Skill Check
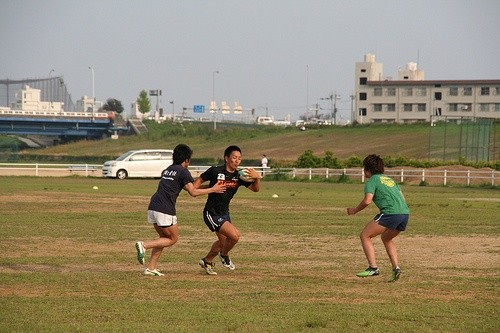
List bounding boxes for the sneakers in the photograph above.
[135,241,146,265]
[199,257,218,275]
[388,266,402,282]
[218,251,236,270]
[143,267,165,277]
[356,267,380,278]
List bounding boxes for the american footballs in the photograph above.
[237,168,256,182]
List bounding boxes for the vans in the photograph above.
[102,149,173,180]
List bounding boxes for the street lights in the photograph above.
[47,69,55,113]
[88,65,94,120]
[306,64,311,121]
[212,71,220,120]
[170,100,175,121]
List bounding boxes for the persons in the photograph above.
[136,143,227,277]
[347,154,409,283]
[261,154,268,168]
[300,126,306,131]
[195,145,261,276]
